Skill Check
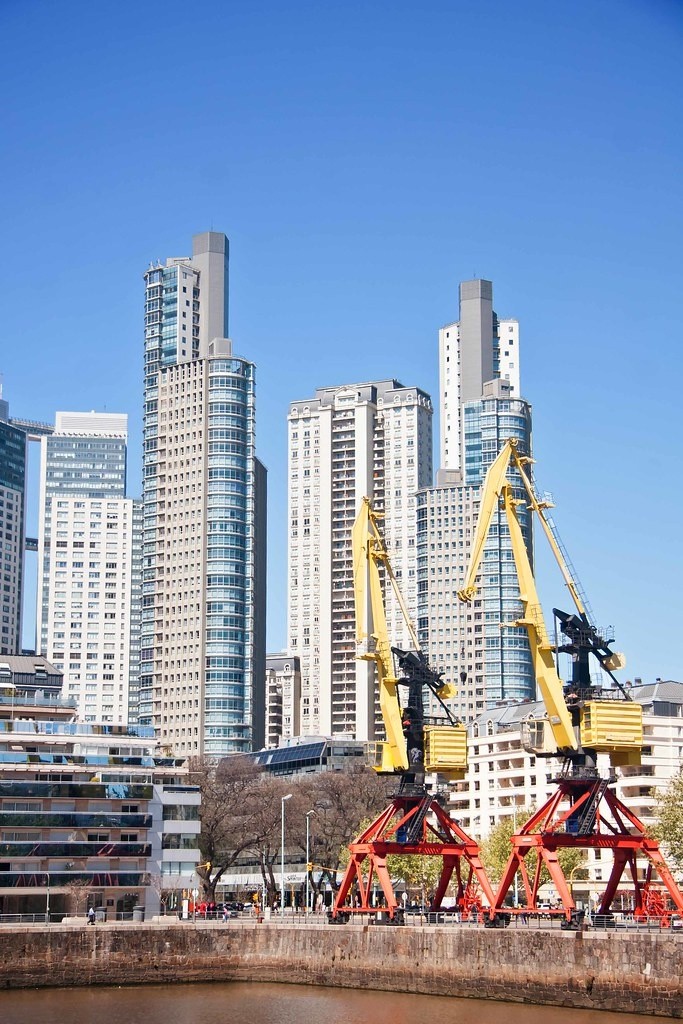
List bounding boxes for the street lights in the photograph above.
[304,809,315,920]
[280,793,294,920]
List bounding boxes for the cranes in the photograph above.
[456,437,683,930]
[326,494,512,928]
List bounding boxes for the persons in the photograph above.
[221,904,228,923]
[272,899,279,913]
[86,906,95,925]
[517,903,527,925]
